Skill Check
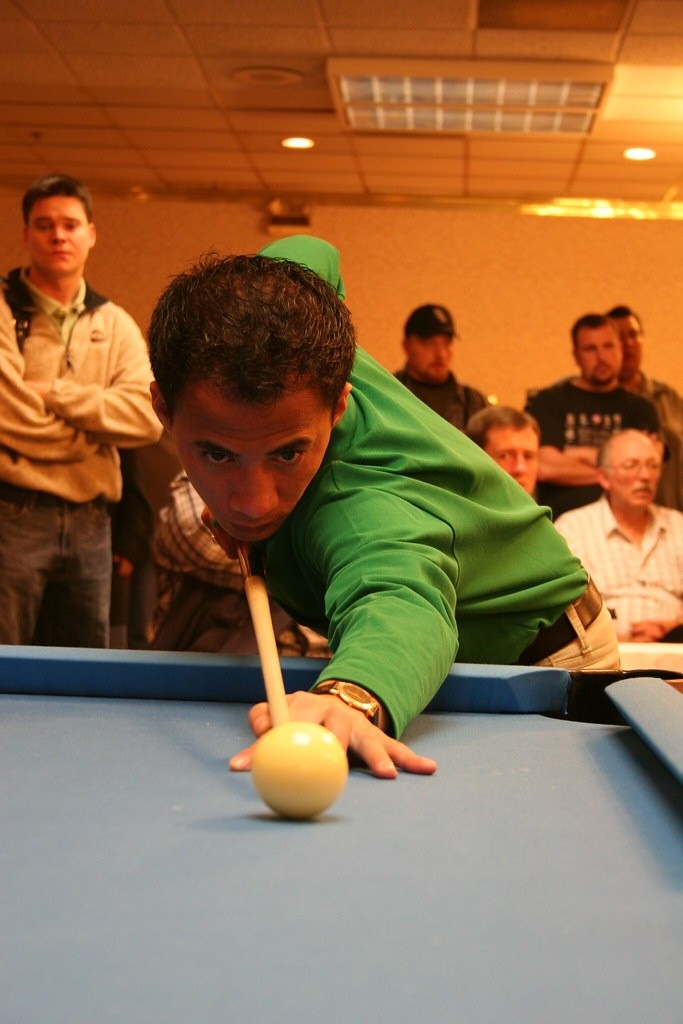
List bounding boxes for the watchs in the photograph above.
[312,682,380,726]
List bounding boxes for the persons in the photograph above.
[148,234,625,783]
[0,172,683,671]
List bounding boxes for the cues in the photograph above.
[236,539,291,732]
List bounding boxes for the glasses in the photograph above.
[603,459,667,477]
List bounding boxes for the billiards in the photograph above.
[251,720,350,821]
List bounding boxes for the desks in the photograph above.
[0,647,683,1024]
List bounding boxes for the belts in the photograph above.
[517,577,602,666]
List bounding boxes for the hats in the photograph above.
[404,305,460,341]
